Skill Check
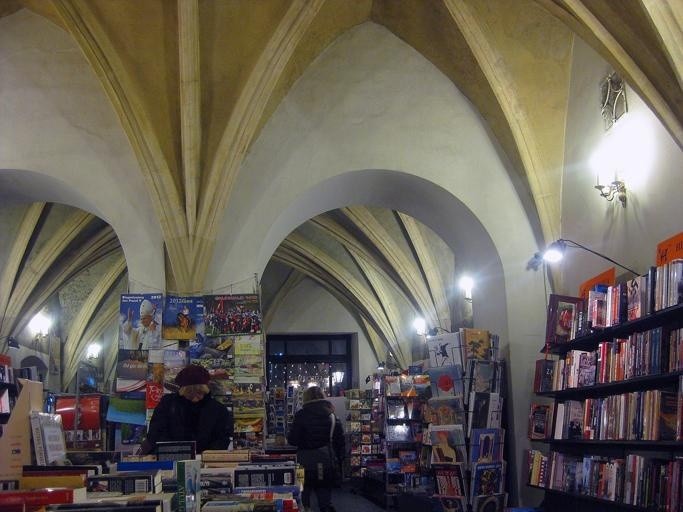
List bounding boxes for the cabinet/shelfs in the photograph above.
[343,302,682,512]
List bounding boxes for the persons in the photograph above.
[123,300,159,349]
[148,363,210,453]
[287,386,345,512]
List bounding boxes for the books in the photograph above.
[1,366,39,413]
[88,441,201,511]
[266,387,303,440]
[31,412,66,465]
[347,328,508,512]
[1,462,117,510]
[527,258,683,511]
[199,450,305,511]
[107,295,266,454]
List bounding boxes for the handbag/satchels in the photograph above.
[303,446,339,486]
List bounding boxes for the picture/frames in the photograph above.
[545,294,580,343]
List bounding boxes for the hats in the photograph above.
[173,363,211,386]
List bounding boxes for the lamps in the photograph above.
[600,69,628,132]
[544,239,641,276]
